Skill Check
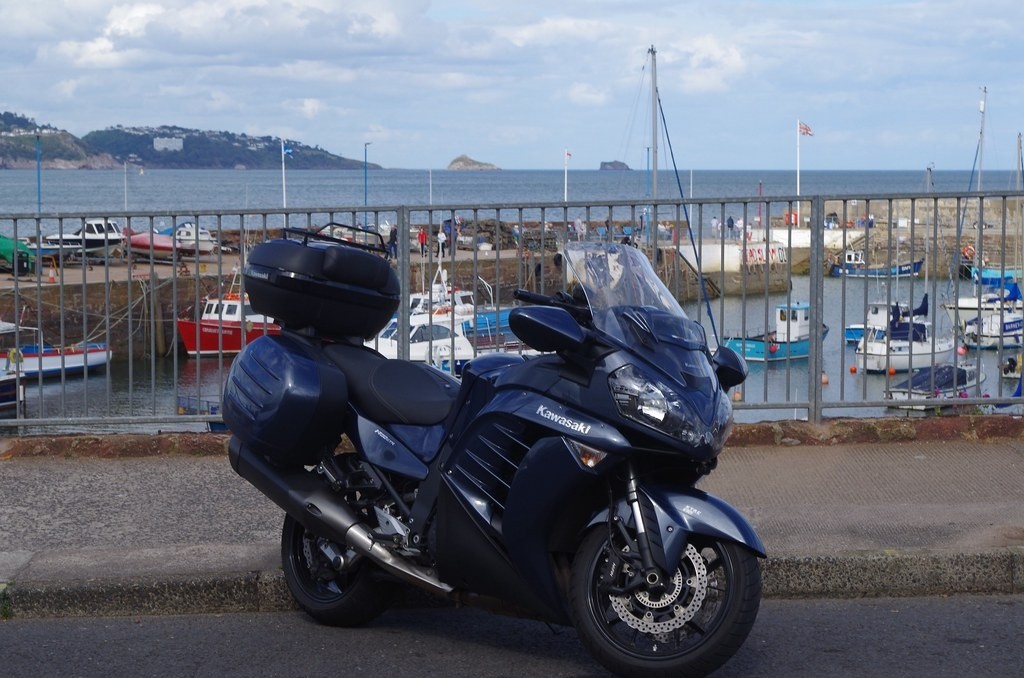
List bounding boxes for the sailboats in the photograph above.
[950,132,1023,351]
[940,128,1023,341]
[313,167,560,375]
[156,220,218,258]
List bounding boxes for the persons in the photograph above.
[418,227,427,257]
[727,216,733,232]
[710,216,718,239]
[435,229,446,258]
[388,224,397,259]
[575,213,583,241]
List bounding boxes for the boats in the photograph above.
[723,298,830,372]
[45,218,128,262]
[121,225,182,263]
[0,231,37,277]
[0,294,113,384]
[844,324,886,346]
[828,239,925,280]
[882,356,987,411]
[855,199,956,375]
[177,218,283,359]
[970,264,1023,285]
[998,351,1024,381]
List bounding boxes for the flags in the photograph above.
[799,122,813,136]
[283,146,294,159]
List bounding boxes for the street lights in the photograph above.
[646,146,651,250]
[365,143,374,245]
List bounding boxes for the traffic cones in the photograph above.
[44,262,59,284]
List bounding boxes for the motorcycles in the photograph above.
[224,222,768,678]
